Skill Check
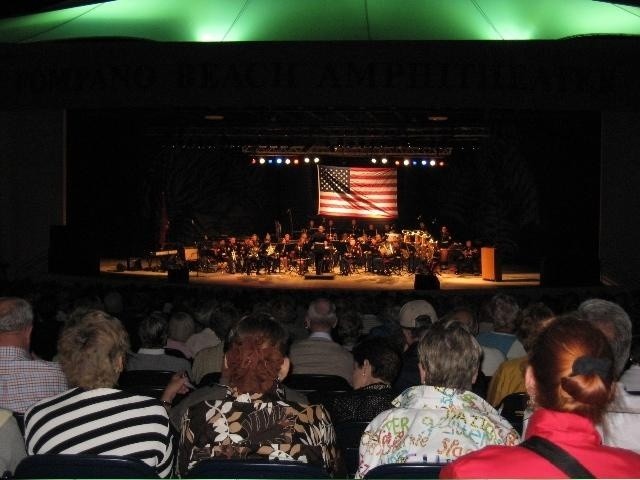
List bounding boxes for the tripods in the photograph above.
[288,243,310,277]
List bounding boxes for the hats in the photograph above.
[397,299,439,330]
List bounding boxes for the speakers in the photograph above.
[414,274,440,289]
[168,268,189,283]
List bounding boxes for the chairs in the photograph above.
[333,418,370,480]
[11,453,160,480]
[281,373,351,403]
[362,462,448,480]
[495,392,530,437]
[198,371,222,388]
[181,457,329,479]
[116,369,178,398]
[11,411,25,435]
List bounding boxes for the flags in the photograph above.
[317,161,401,219]
